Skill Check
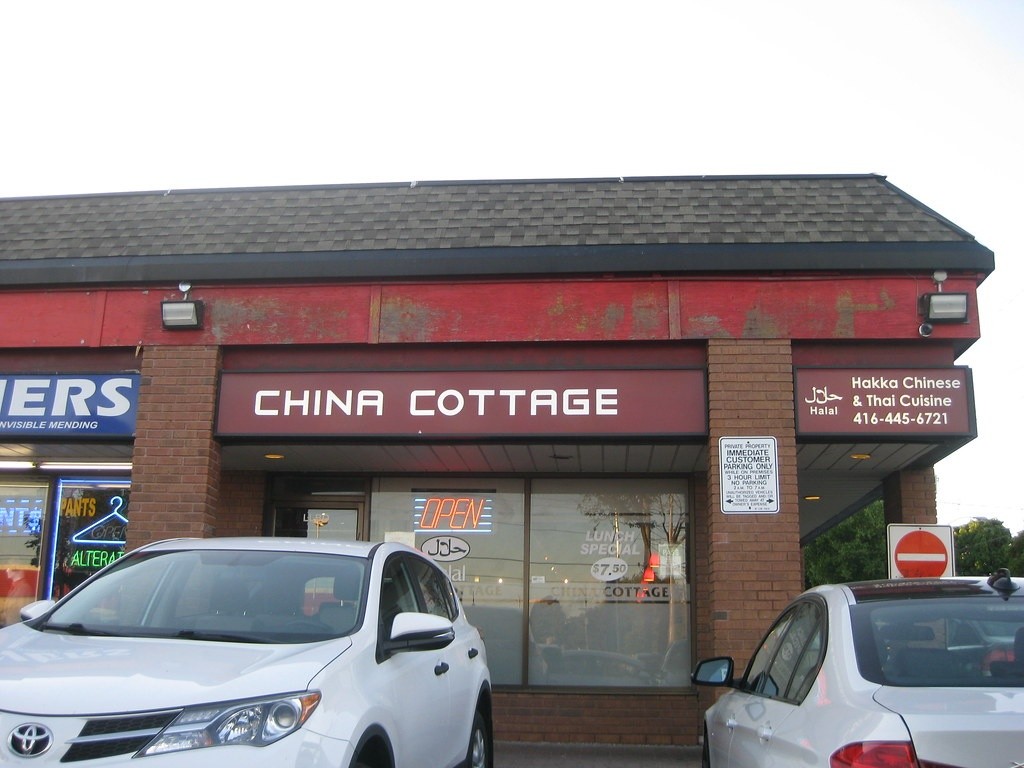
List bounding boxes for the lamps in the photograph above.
[160,279,204,329]
[918,270,969,324]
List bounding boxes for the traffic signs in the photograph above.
[718,436,780,515]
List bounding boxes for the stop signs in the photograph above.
[887,524,955,579]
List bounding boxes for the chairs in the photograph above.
[317,566,361,633]
[197,584,251,632]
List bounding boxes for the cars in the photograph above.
[464,605,542,685]
[0,537,494,768]
[660,640,691,688]
[544,648,655,687]
[691,568,1024,768]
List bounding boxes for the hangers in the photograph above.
[73,496,128,544]
